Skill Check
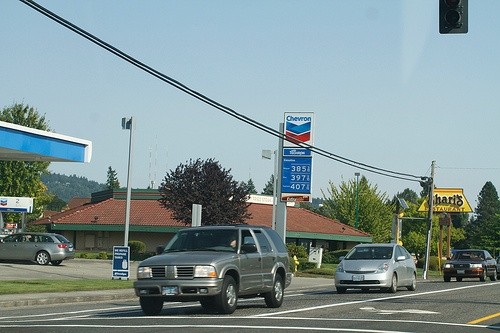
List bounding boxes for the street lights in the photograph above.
[121,115,133,246]
[355,172,360,229]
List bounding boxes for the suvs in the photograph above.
[0,228,76,266]
[133,223,294,316]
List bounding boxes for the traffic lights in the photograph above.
[438,0,468,34]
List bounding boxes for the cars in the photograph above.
[334,242,417,294]
[496,255,500,280]
[442,248,498,283]
